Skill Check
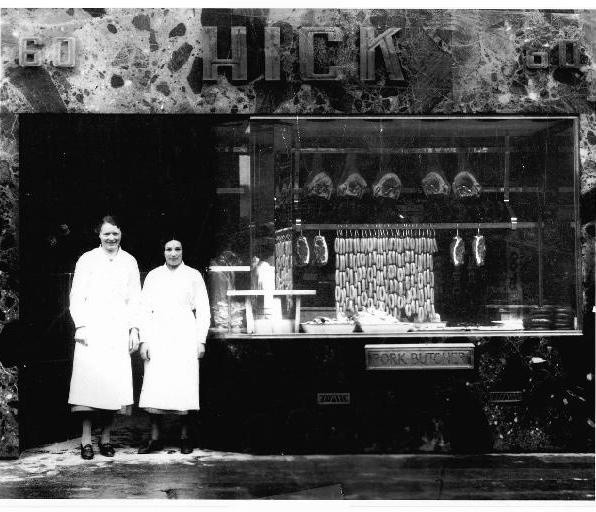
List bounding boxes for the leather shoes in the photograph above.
[81,443,94,459]
[138,439,163,454]
[98,440,114,456]
[182,438,192,454]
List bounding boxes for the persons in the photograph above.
[66,215,142,460]
[135,238,211,456]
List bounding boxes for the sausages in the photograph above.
[334,236,438,322]
[275,241,293,290]
